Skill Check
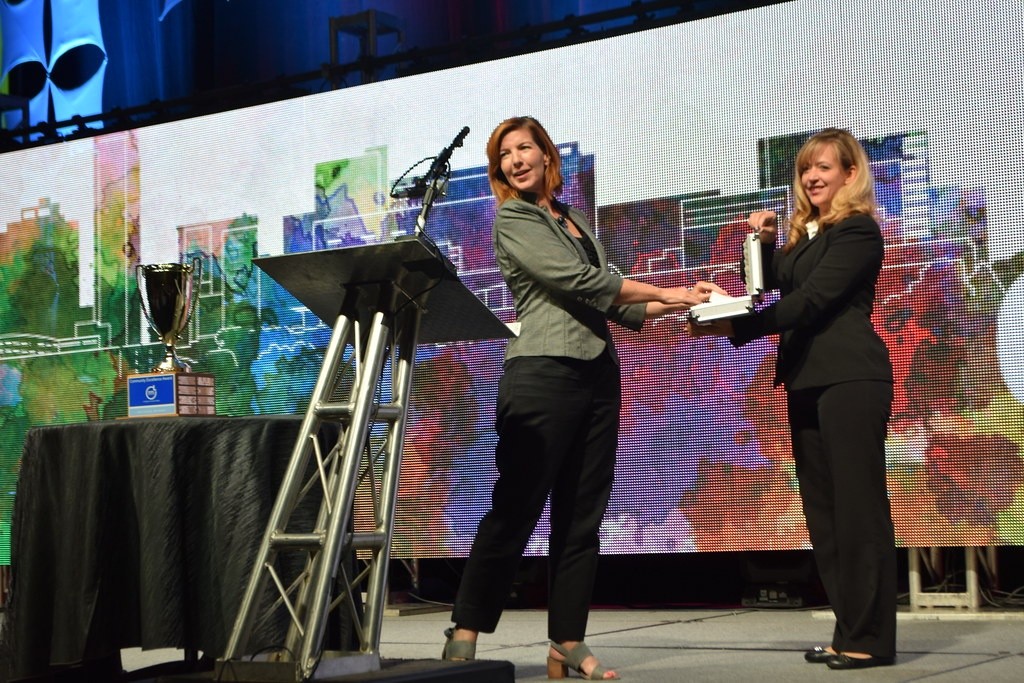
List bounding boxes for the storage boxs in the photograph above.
[127,372,217,418]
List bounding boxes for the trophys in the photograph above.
[134,257,203,374]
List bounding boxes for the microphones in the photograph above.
[393,180,447,198]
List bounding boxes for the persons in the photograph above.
[686,128,899,671]
[437,114,731,680]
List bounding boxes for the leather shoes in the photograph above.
[826,654,895,669]
[805,647,839,663]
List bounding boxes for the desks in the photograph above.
[0,413,365,683]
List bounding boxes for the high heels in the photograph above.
[442,627,476,662]
[547,639,620,680]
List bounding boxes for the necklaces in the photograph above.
[537,202,568,226]
